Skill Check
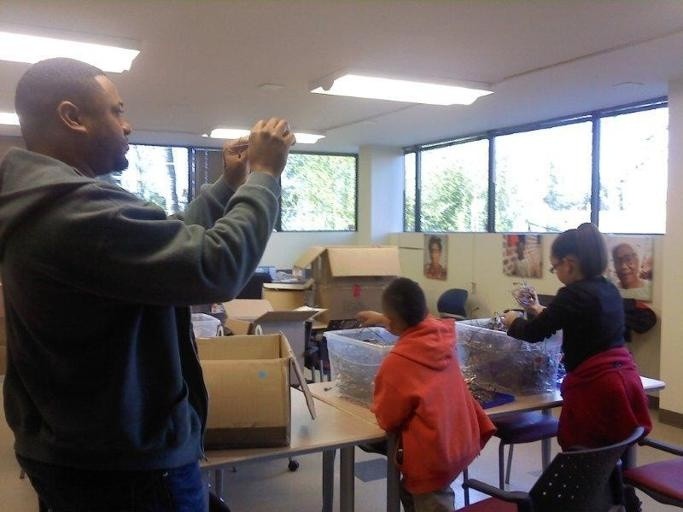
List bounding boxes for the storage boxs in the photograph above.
[197,332,316,450]
[260,279,314,312]
[294,244,400,328]
[222,299,328,386]
[323,326,400,407]
[455,318,564,394]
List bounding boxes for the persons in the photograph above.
[1,58,297,512]
[499,222,653,512]
[613,242,653,302]
[424,235,447,281]
[354,278,497,512]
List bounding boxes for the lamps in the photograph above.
[0,31,140,74]
[201,128,326,144]
[309,68,496,106]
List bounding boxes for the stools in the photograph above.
[619,439,683,512]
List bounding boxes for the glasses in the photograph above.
[614,254,634,267]
[549,259,572,273]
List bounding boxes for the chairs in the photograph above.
[436,288,468,321]
